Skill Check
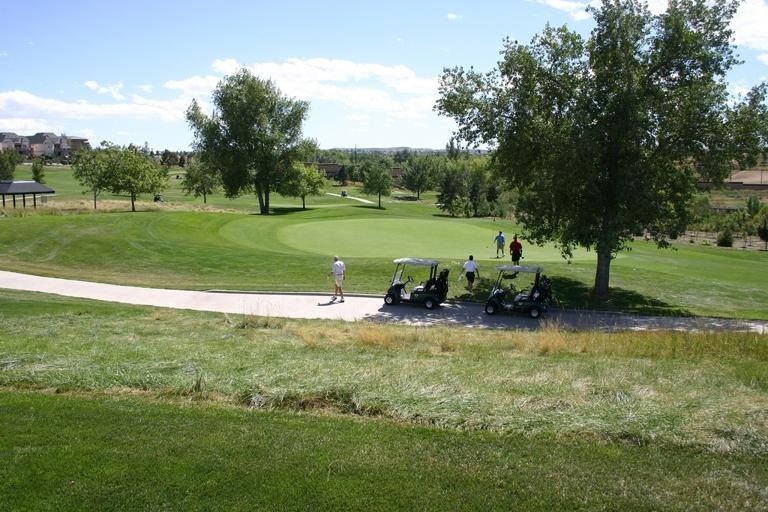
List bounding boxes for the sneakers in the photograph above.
[338,298,345,303]
[329,296,337,304]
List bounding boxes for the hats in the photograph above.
[333,255,339,260]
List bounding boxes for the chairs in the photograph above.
[412,280,432,293]
[513,284,537,302]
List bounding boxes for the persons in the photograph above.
[509,235,523,266]
[326,255,346,303]
[459,255,479,292]
[493,230,505,258]
